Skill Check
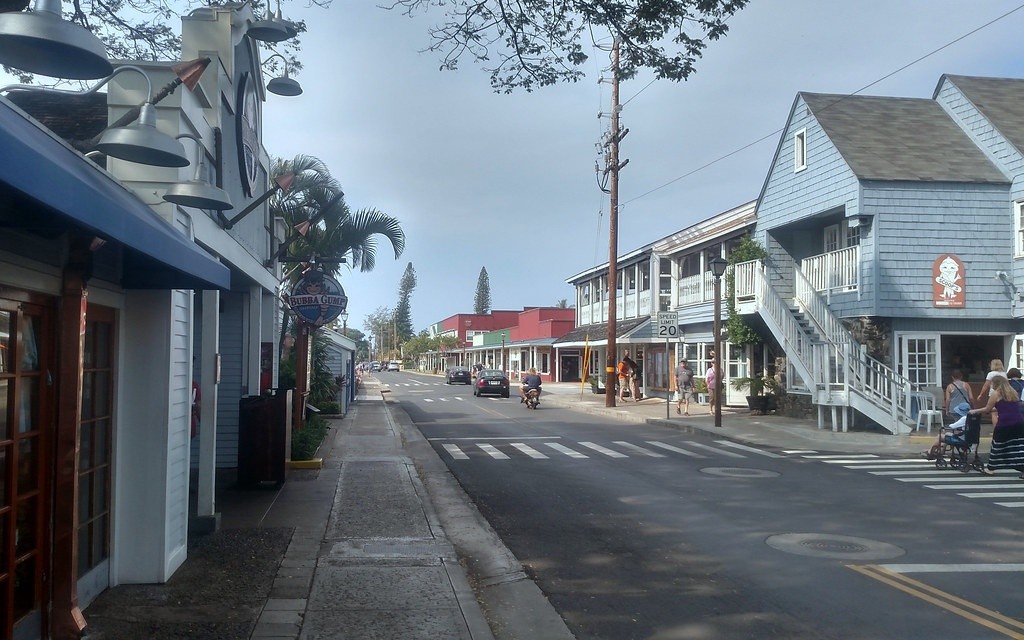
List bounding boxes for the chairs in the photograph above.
[914,390,945,433]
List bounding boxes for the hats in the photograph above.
[954,403,971,417]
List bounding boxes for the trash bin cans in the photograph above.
[243,387,293,485]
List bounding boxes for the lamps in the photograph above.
[84,134,233,212]
[260,53,302,96]
[256,0,298,39]
[0,0,114,81]
[235,0,288,44]
[0,63,192,169]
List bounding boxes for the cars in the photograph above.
[472,369,510,398]
[355,361,400,372]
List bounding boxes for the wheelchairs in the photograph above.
[926,413,984,472]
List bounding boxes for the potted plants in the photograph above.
[729,377,768,411]
[588,377,598,393]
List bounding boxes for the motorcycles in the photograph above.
[521,376,541,408]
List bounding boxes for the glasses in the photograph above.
[681,361,686,362]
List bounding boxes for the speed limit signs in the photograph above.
[657,311,677,338]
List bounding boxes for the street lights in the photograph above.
[341,309,349,337]
[368,335,372,377]
[501,333,506,373]
[709,256,729,427]
[463,341,466,367]
[445,366,471,385]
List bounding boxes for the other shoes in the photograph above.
[619,397,723,417]
[982,468,994,476]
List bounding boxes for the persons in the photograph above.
[673,357,696,417]
[519,367,542,405]
[967,374,1024,480]
[920,402,973,460]
[1006,367,1024,402]
[618,357,631,403]
[705,358,725,416]
[474,361,483,371]
[627,361,644,403]
[945,369,974,422]
[976,358,1008,428]
